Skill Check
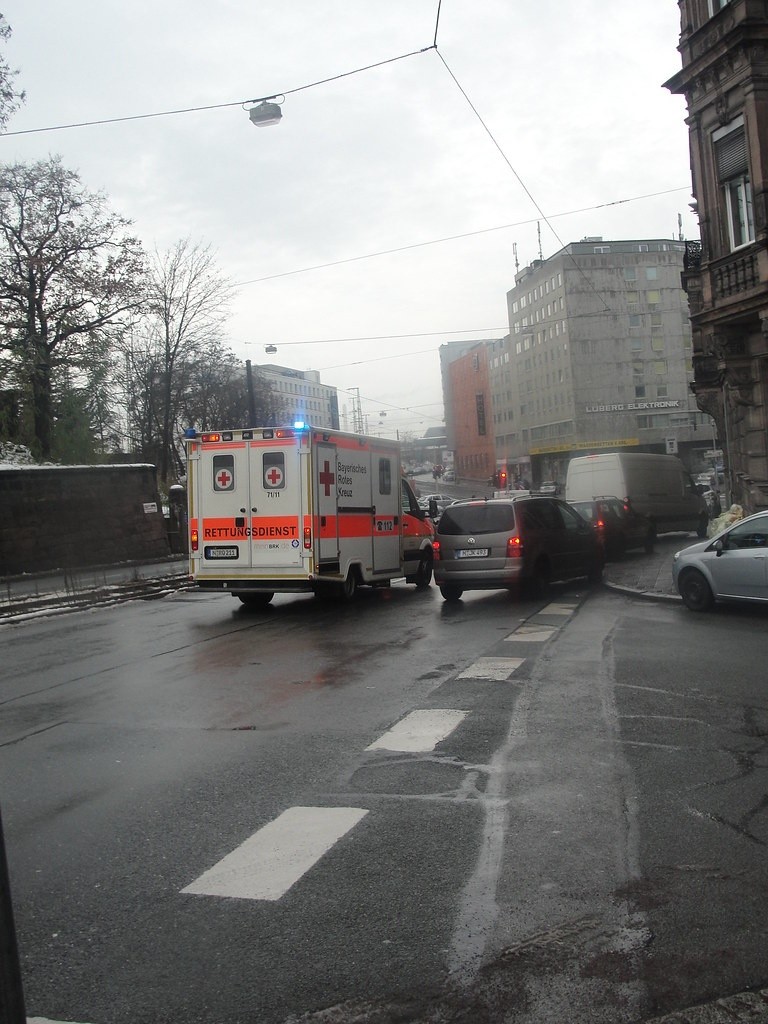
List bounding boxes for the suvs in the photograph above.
[564,496,658,562]
[431,490,600,601]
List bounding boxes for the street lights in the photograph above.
[711,420,720,494]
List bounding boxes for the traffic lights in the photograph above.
[502,473,506,478]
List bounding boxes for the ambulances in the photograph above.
[184,421,438,606]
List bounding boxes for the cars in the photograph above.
[672,510,768,612]
[539,481,561,495]
[443,471,454,481]
[401,501,443,516]
[686,482,722,521]
[564,453,709,538]
[418,494,459,507]
